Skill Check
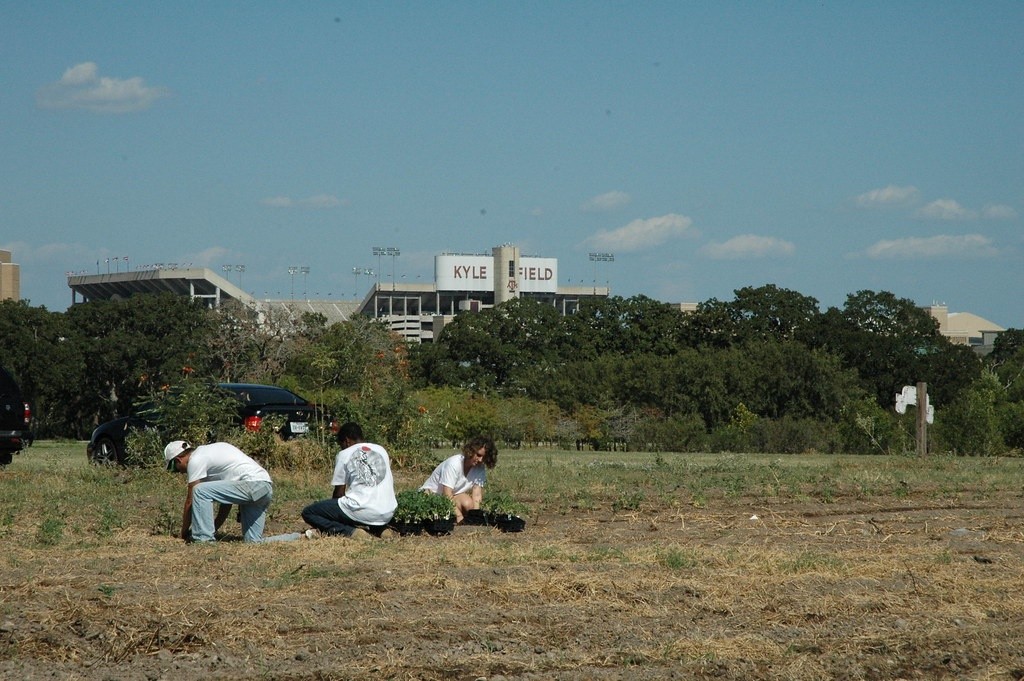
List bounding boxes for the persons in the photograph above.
[301,419,401,543]
[419,437,499,525]
[164,440,318,544]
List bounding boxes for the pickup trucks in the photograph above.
[0,391,35,466]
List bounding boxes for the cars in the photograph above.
[88,382,337,468]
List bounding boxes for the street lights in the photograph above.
[589,251,601,295]
[288,265,297,299]
[352,267,361,301]
[300,266,309,299]
[235,265,245,290]
[222,264,231,282]
[602,253,614,298]
[372,246,387,290]
[364,268,374,292]
[386,248,400,290]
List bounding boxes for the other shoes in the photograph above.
[306,528,320,540]
[381,528,396,539]
[351,528,372,539]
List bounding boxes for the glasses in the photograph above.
[169,460,178,473]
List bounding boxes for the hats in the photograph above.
[164,441,191,470]
